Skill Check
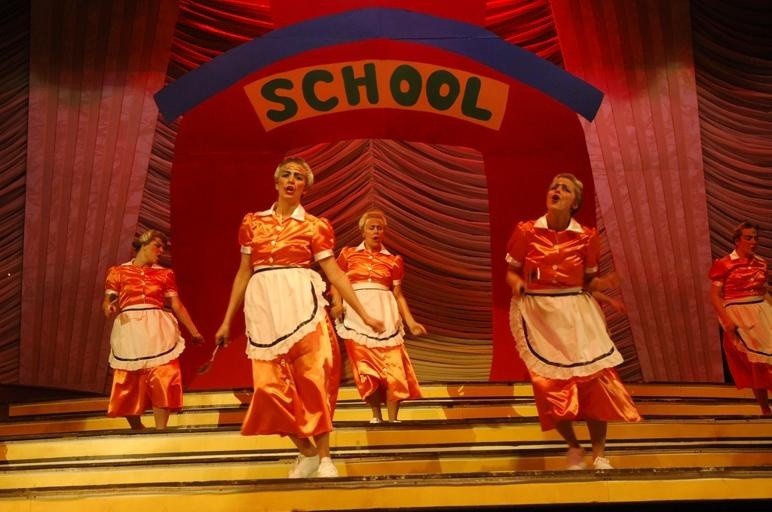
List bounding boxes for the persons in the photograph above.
[707,219,772,420]
[503,168,644,469]
[104,229,204,428]
[329,207,429,422]
[214,156,385,479]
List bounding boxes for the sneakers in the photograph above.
[568,448,585,470]
[288,454,338,479]
[593,457,614,469]
